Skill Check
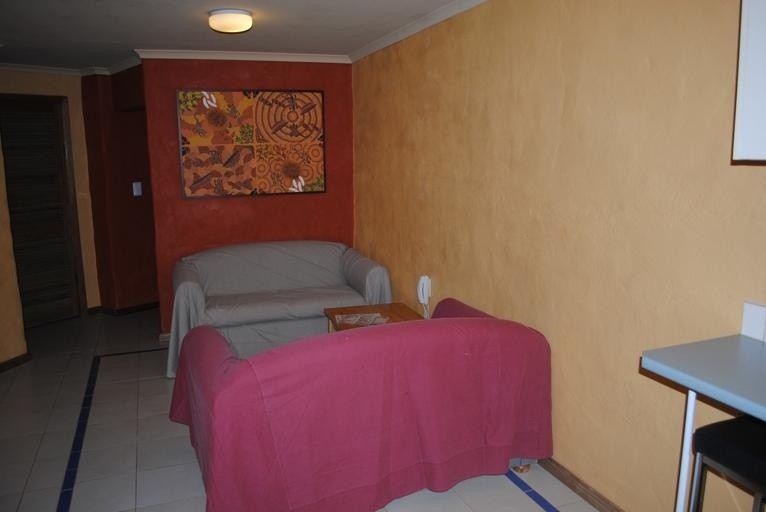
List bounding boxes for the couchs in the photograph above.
[171,296,553,512]
[171,241,410,377]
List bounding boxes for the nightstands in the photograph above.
[322,296,431,334]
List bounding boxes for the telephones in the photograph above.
[416,275,431,305]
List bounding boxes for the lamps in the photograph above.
[207,9,253,33]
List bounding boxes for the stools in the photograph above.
[689,414,765,511]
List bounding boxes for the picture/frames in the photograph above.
[175,86,327,201]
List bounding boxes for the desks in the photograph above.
[637,334,766,511]
